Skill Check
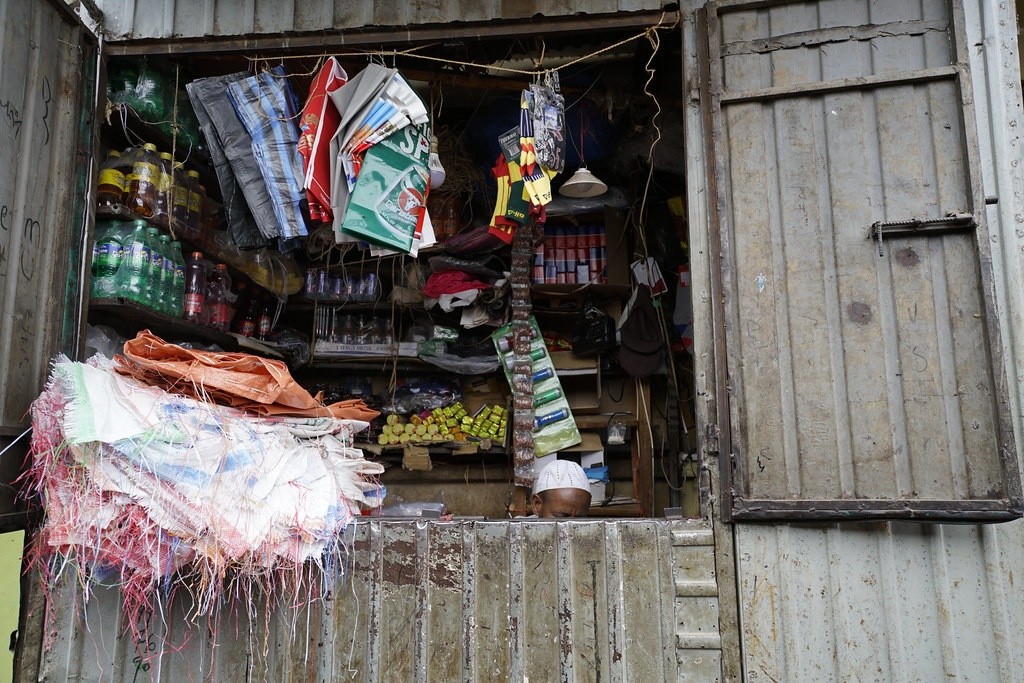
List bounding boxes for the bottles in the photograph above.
[328,315,393,344]
[97,143,202,230]
[90,58,206,145]
[90,221,272,340]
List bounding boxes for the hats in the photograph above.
[532,460,592,496]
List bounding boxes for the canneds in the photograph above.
[303,268,378,296]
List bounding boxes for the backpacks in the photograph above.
[617,283,666,379]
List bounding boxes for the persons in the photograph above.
[513,460,593,519]
